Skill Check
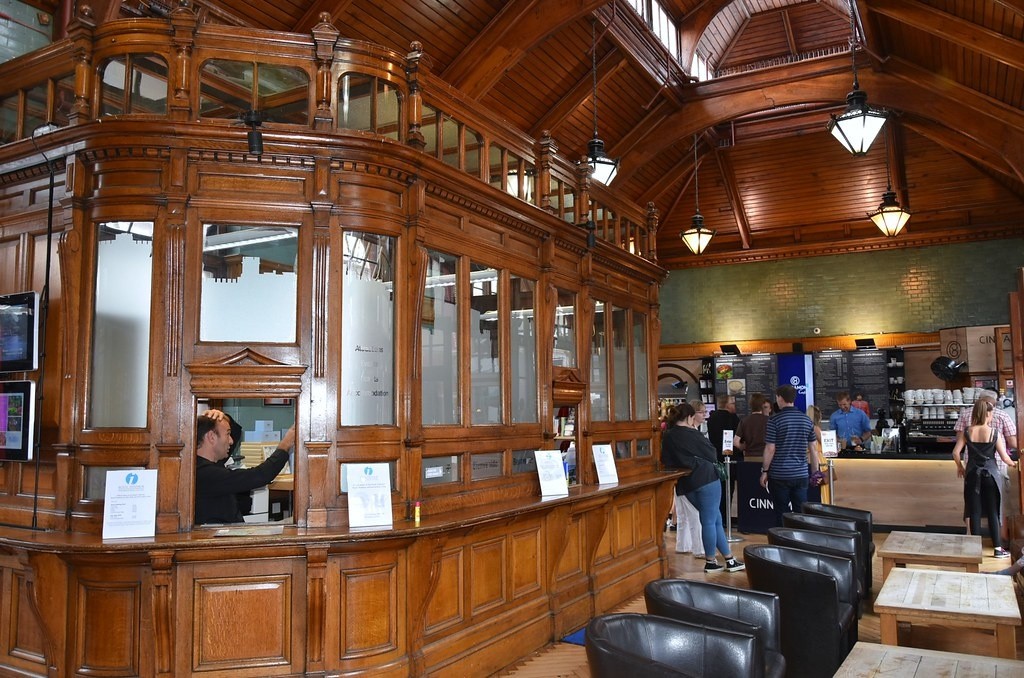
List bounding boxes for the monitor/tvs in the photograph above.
[0,380,36,462]
[0,291,39,373]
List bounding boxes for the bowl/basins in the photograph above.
[903,386,989,419]
[728,380,743,394]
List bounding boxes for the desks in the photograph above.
[877,531,983,573]
[833,641,1024,678]
[872,566,1022,660]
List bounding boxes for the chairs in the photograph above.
[585,500,876,678]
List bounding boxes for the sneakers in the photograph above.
[725,556,745,572]
[670,523,677,531]
[994,547,1010,557]
[704,559,724,572]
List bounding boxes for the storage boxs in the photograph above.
[244,420,281,442]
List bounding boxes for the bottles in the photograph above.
[870,442,882,454]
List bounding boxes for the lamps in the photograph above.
[824,0,890,158]
[576,221,596,248]
[576,17,621,187]
[679,134,719,255]
[929,356,965,382]
[232,115,263,156]
[866,115,913,240]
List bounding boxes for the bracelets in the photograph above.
[860,437,864,442]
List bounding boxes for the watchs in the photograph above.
[761,467,769,473]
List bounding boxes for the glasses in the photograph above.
[696,410,708,415]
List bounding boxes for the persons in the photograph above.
[851,393,870,418]
[807,405,831,505]
[708,396,740,528]
[760,385,822,528]
[952,390,1018,558]
[733,394,781,452]
[659,400,745,574]
[829,392,871,450]
[195,409,296,525]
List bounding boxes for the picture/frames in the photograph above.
[0,380,36,462]
[262,398,294,408]
[0,290,40,375]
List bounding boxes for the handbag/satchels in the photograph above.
[821,467,837,485]
